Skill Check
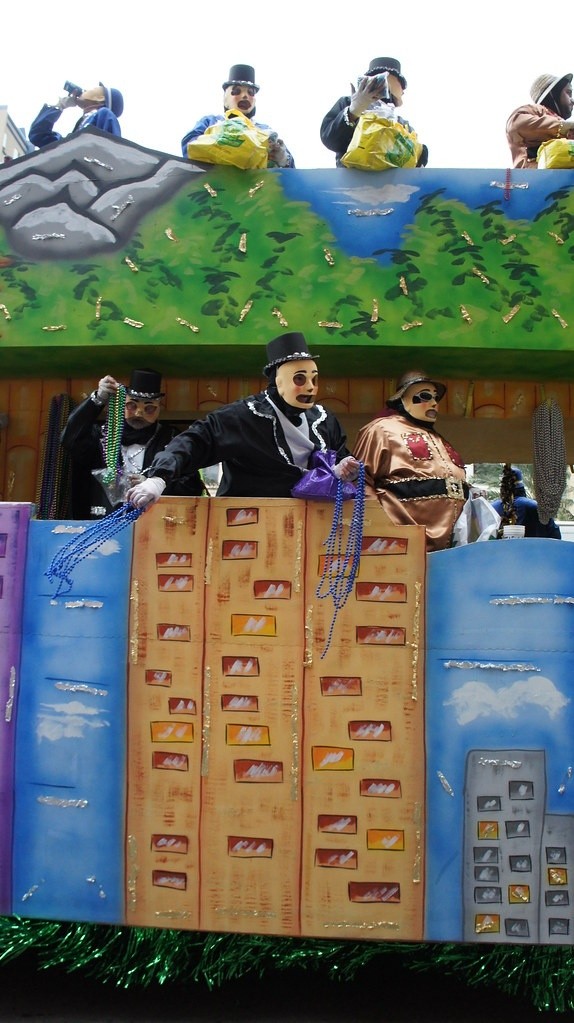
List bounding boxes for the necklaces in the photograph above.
[103,384,127,485]
[35,393,78,521]
[44,502,144,600]
[532,401,567,525]
[316,462,366,659]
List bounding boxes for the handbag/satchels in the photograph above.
[340,110,423,172]
[534,138,574,169]
[289,448,359,502]
[450,489,502,550]
[186,108,270,171]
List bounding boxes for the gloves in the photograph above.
[349,77,387,118]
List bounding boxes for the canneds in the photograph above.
[63,80,82,96]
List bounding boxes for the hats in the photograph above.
[98,81,124,118]
[363,56,407,91]
[121,367,166,401]
[261,331,320,376]
[221,63,260,93]
[529,72,573,106]
[499,464,526,489]
[386,370,447,410]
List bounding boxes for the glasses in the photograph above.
[414,391,441,404]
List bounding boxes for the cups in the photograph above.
[503,524,525,539]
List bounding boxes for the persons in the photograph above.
[28,81,123,148]
[506,73,574,168]
[126,332,359,511]
[320,57,428,168]
[352,369,487,552]
[490,468,561,540]
[60,368,211,522]
[181,65,295,168]
